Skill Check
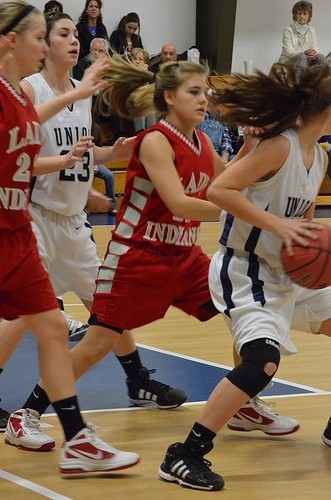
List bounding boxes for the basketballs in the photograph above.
[280,221,331,290]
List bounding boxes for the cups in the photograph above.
[244,61,253,75]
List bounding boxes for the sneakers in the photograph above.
[59,422,142,473]
[158,443,225,490]
[56,297,90,341]
[321,418,331,445]
[125,367,187,409]
[0,408,11,431]
[4,407,56,451]
[226,381,300,436]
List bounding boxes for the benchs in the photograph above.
[86,75,243,214]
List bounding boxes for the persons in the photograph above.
[195,62,288,161]
[56,296,89,341]
[132,48,149,69]
[73,0,107,57]
[109,12,143,56]
[0,0,141,478]
[148,44,177,74]
[1,10,187,409]
[157,65,331,492]
[279,0,328,83]
[44,0,63,13]
[74,38,110,80]
[6,56,300,450]
